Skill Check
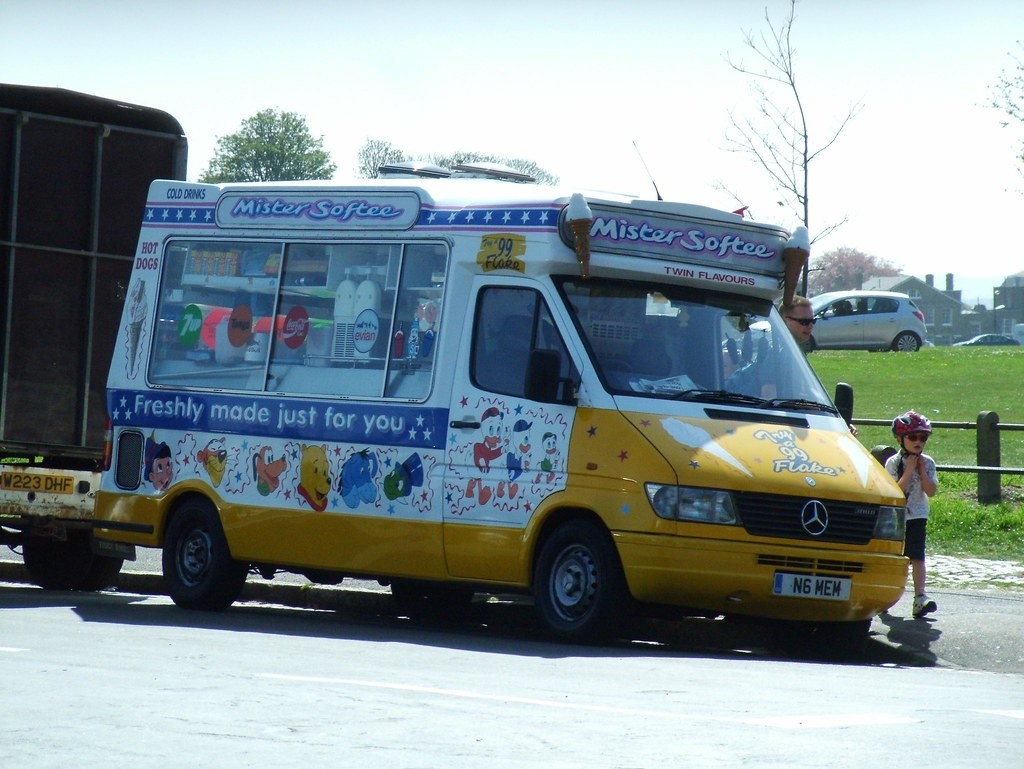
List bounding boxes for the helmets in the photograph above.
[891,411,934,436]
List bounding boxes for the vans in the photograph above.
[90,175,912,637]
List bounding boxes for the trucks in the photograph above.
[0,82,189,590]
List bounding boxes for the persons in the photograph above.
[758,296,857,435]
[884,410,937,617]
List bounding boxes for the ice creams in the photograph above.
[565,192,594,279]
[784,226,811,305]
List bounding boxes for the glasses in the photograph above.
[786,315,818,327]
[901,431,930,441]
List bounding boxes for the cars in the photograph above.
[953,334,1021,346]
[796,289,929,352]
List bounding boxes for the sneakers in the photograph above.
[913,595,938,617]
